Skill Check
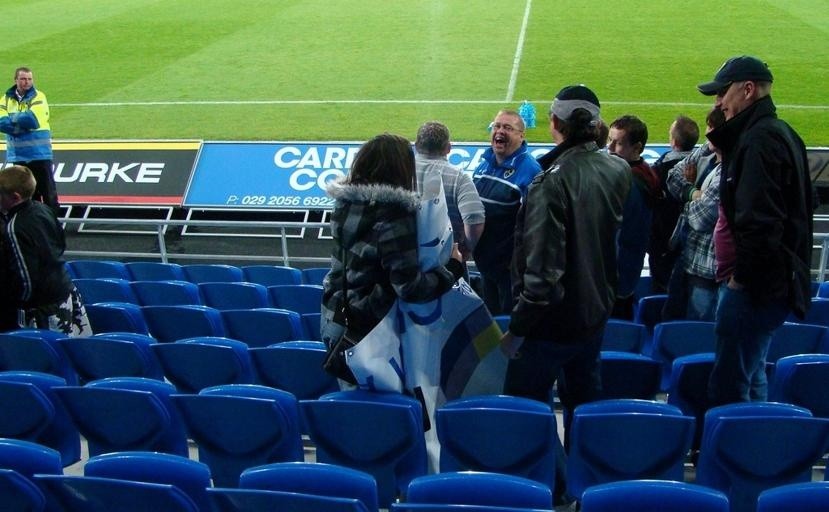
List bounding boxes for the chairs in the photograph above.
[139,304,230,342]
[564,399,698,498]
[267,284,325,314]
[0,329,81,387]
[638,295,699,354]
[56,332,165,383]
[435,395,568,506]
[32,448,213,512]
[247,339,340,397]
[600,351,660,403]
[241,264,304,285]
[769,320,829,367]
[817,281,829,298]
[149,336,260,394]
[663,351,776,450]
[492,314,558,412]
[599,318,648,356]
[86,301,149,336]
[634,276,657,307]
[52,378,190,459]
[810,280,821,297]
[169,384,305,489]
[130,279,205,309]
[390,471,553,512]
[705,401,829,511]
[218,305,306,349]
[804,296,829,331]
[0,371,82,468]
[181,263,249,287]
[68,260,131,281]
[579,480,730,512]
[214,461,380,512]
[302,312,324,344]
[652,320,721,393]
[300,392,429,510]
[73,277,139,306]
[302,267,332,285]
[197,281,274,309]
[0,438,64,512]
[759,483,829,512]
[768,351,829,419]
[124,261,189,284]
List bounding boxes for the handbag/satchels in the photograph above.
[321,249,367,386]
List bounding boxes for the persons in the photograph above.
[0,68,61,215]
[698,57,813,404]
[319,134,463,391]
[609,115,663,318]
[590,119,608,152]
[713,191,740,320]
[662,107,724,320]
[0,166,93,338]
[472,112,542,318]
[502,85,632,509]
[412,121,487,275]
[652,116,702,295]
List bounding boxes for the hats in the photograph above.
[550,86,601,126]
[696,56,774,96]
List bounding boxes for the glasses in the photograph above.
[493,124,521,133]
